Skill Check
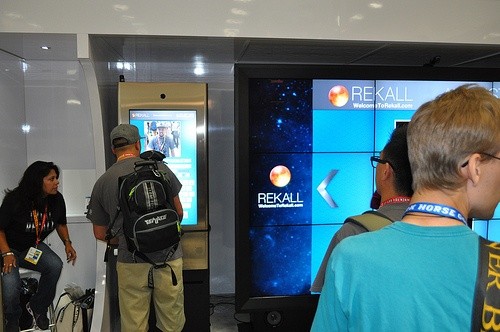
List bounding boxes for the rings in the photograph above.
[4,264,5,265]
[9,264,12,266]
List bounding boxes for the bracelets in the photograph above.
[63,239,72,245]
[2,251,14,256]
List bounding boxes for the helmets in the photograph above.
[157,122,168,127]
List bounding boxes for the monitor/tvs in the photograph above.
[129,108,197,225]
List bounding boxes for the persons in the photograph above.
[0,160,76,332]
[311,84,500,332]
[86,124,185,332]
[146,125,175,157]
[312,122,414,291]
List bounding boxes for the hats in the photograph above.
[110,124,145,148]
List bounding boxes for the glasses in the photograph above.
[371,156,396,176]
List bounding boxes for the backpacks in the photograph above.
[114,159,184,252]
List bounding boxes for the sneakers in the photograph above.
[26,297,49,330]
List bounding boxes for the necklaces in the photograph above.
[406,213,440,217]
